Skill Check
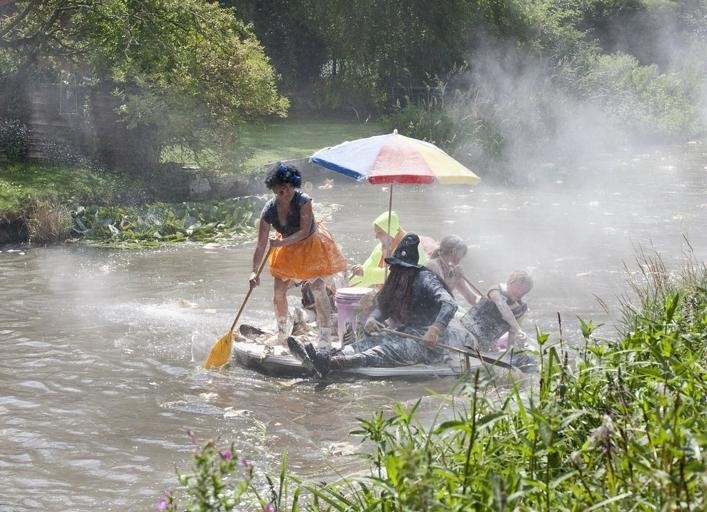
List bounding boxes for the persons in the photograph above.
[426,235,481,308]
[248,161,347,345]
[347,210,426,289]
[460,271,534,353]
[288,232,456,380]
[286,273,336,337]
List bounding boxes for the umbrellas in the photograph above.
[308,128,481,284]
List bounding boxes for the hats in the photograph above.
[385,233,426,270]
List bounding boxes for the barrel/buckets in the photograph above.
[334,288,376,345]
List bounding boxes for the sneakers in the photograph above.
[287,337,313,371]
[304,342,331,377]
[265,333,286,345]
[316,338,331,349]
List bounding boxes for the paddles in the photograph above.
[206,243,274,369]
[374,323,518,374]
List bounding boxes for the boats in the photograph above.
[229,315,509,382]
